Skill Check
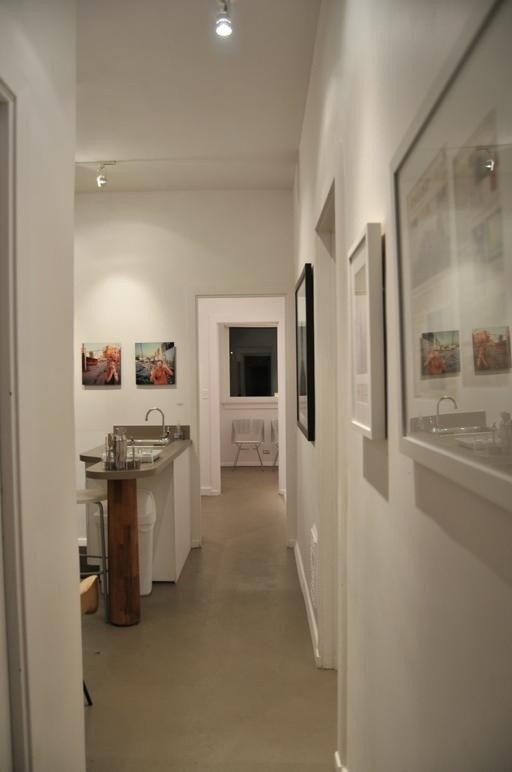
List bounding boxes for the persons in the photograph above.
[149,354,174,384]
[103,359,121,385]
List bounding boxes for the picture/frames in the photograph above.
[406,101,506,301]
[346,223,386,442]
[386,5,512,513]
[294,261,315,443]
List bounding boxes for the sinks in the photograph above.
[126,439,168,448]
[432,425,496,436]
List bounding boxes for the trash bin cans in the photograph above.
[92,491,158,596]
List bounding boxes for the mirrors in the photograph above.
[222,323,281,405]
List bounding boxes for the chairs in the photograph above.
[230,419,266,473]
[78,574,101,706]
[270,418,279,471]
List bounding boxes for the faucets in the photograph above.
[436,395,458,425]
[145,407,169,439]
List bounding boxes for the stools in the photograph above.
[76,488,108,611]
